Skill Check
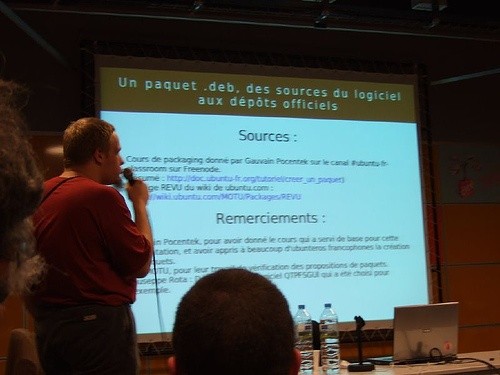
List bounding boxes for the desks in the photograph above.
[333,349,500,375]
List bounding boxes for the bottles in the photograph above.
[319,303,340,375]
[293,305,314,375]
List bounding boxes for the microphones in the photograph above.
[123,167,133,187]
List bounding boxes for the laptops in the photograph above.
[367,301,460,365]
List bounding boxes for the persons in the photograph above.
[22,117,153,375]
[168,268,302,375]
